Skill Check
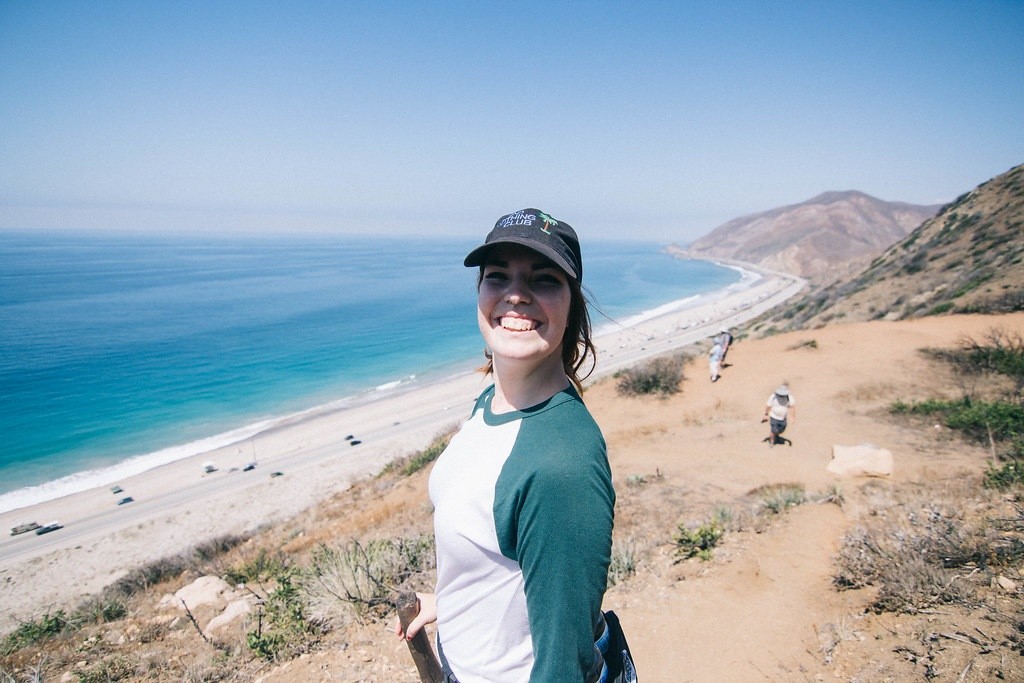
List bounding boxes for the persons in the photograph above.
[396,208,639,683]
[761,385,795,448]
[709,327,730,382]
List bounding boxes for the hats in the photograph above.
[713,337,720,344]
[720,328,728,332]
[464,208,582,285]
[775,385,790,397]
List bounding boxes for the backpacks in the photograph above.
[727,334,733,346]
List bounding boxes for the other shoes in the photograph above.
[711,377,716,381]
